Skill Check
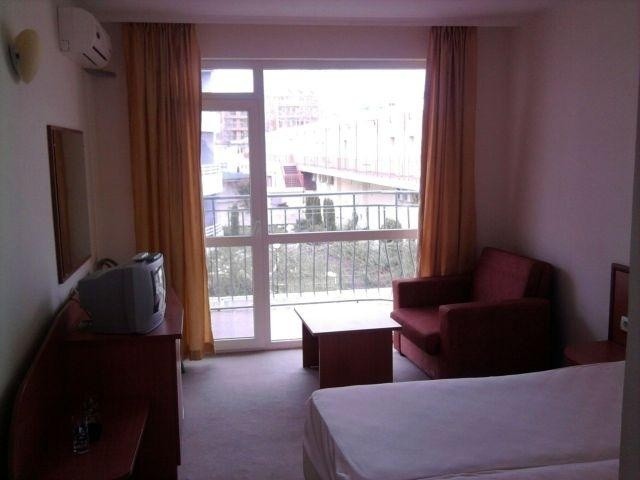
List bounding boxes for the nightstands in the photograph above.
[565,340,626,364]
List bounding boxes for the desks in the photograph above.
[5,286,183,479]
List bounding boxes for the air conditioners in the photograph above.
[55,6,114,70]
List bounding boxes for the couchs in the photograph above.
[389,246,557,379]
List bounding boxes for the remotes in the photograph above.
[132,252,148,261]
[145,252,162,263]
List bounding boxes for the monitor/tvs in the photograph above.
[78,255,166,333]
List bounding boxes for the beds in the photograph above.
[303,262,629,480]
